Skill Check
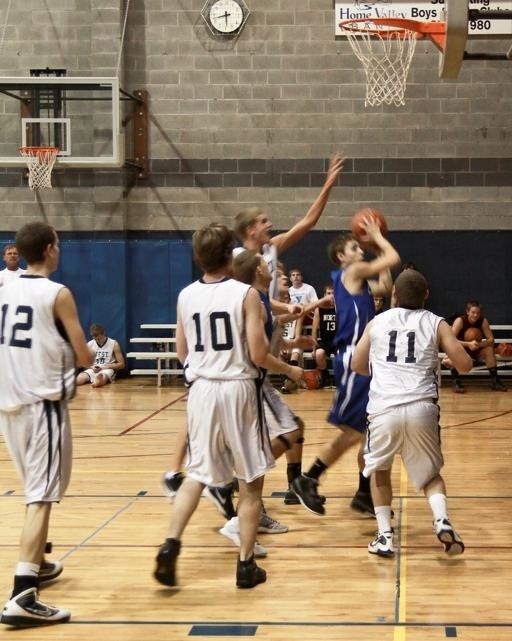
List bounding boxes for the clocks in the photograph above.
[200,0,252,37]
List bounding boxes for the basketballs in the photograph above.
[353,207,387,245]
[464,328,481,341]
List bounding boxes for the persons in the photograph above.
[450,299,509,393]
[0,221,97,627]
[152,152,473,587]
[0,245,26,286]
[75,323,125,388]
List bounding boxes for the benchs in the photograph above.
[127,322,511,387]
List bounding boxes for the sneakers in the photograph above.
[492,375,508,391]
[1,587,71,626]
[284,472,326,515]
[452,378,465,393]
[433,518,464,555]
[368,531,394,555]
[155,542,178,586]
[351,490,394,518]
[39,561,64,582]
[164,472,186,497]
[202,483,289,588]
